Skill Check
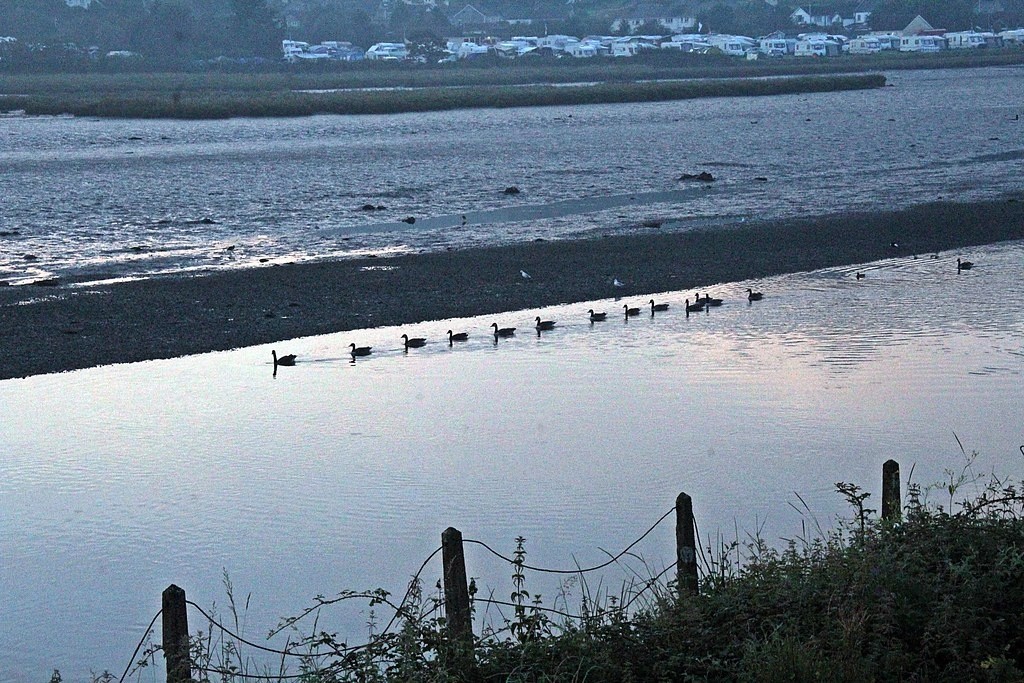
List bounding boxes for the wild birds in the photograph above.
[347,343,372,357]
[587,309,606,321]
[401,335,427,349]
[490,322,516,336]
[649,298,669,311]
[535,317,556,330]
[746,288,764,302]
[956,258,975,271]
[684,299,703,313]
[623,305,641,316]
[856,273,866,280]
[446,331,467,341]
[271,349,298,366]
[695,292,723,307]
[890,240,900,247]
